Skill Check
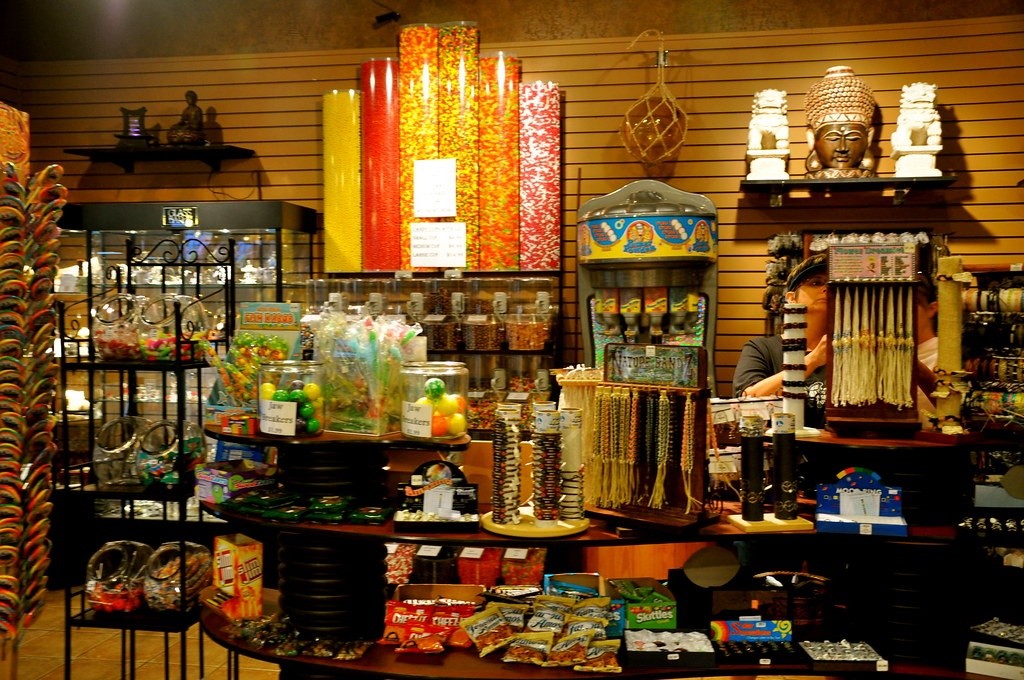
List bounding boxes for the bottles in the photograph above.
[91,292,209,363]
[83,537,212,615]
[92,414,209,494]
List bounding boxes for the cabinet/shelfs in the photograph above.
[3,101,1024,680]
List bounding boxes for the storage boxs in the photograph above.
[213,533,263,621]
[394,583,486,606]
[195,459,278,505]
[605,578,677,630]
[543,572,606,600]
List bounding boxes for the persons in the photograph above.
[730,254,830,398]
[915,273,940,428]
[171,89,202,132]
[804,66,876,180]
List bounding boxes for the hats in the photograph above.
[787,253,828,291]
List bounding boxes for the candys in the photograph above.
[197,334,288,409]
[142,551,213,612]
[90,564,143,613]
[0,162,71,653]
[138,329,207,361]
